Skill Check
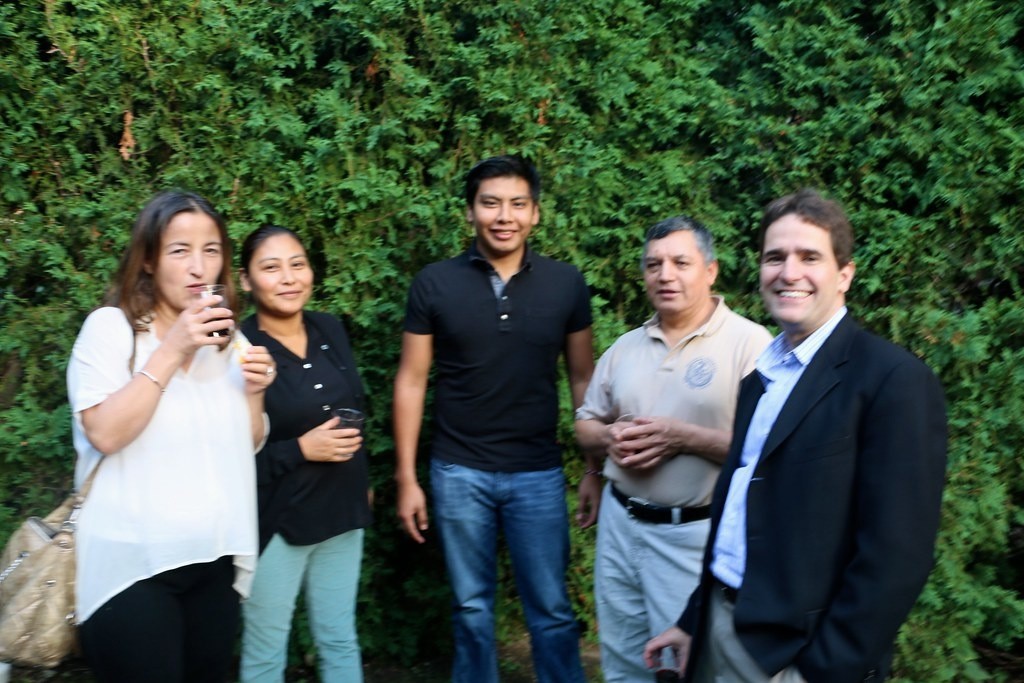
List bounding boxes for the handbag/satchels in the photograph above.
[1,515,80,669]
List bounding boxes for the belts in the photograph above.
[714,578,738,604]
[612,484,713,524]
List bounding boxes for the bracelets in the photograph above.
[585,466,602,477]
[137,370,166,392]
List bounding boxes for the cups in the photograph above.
[192,284,229,337]
[651,646,684,683]
[331,408,366,457]
[613,414,649,455]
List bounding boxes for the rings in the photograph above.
[267,367,273,376]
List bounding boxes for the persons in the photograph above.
[236,224,373,683]
[393,158,602,683]
[66,190,277,683]
[644,189,948,683]
[574,216,775,683]
